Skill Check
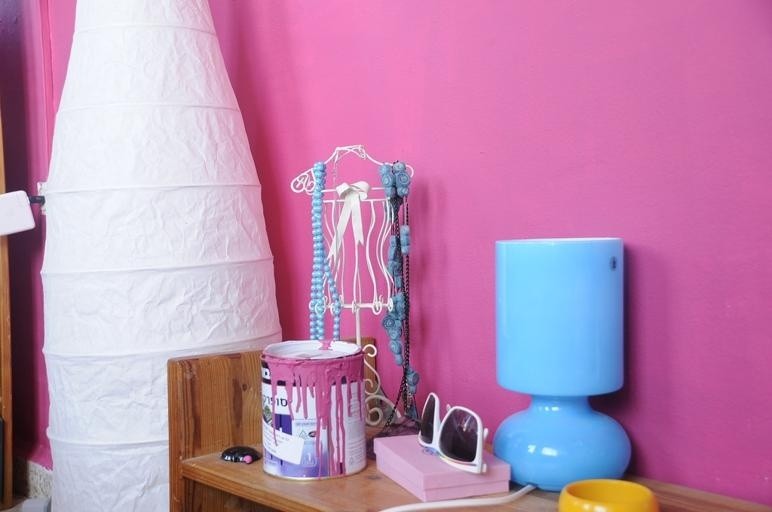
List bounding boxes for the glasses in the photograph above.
[418,391,490,474]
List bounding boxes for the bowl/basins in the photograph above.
[557,478,661,512]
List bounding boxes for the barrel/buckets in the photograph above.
[259,337,369,481]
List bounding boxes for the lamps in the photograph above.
[489,236,633,491]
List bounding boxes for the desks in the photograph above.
[168,336,771,511]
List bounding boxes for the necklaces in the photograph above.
[379,161,420,421]
[308,162,341,342]
